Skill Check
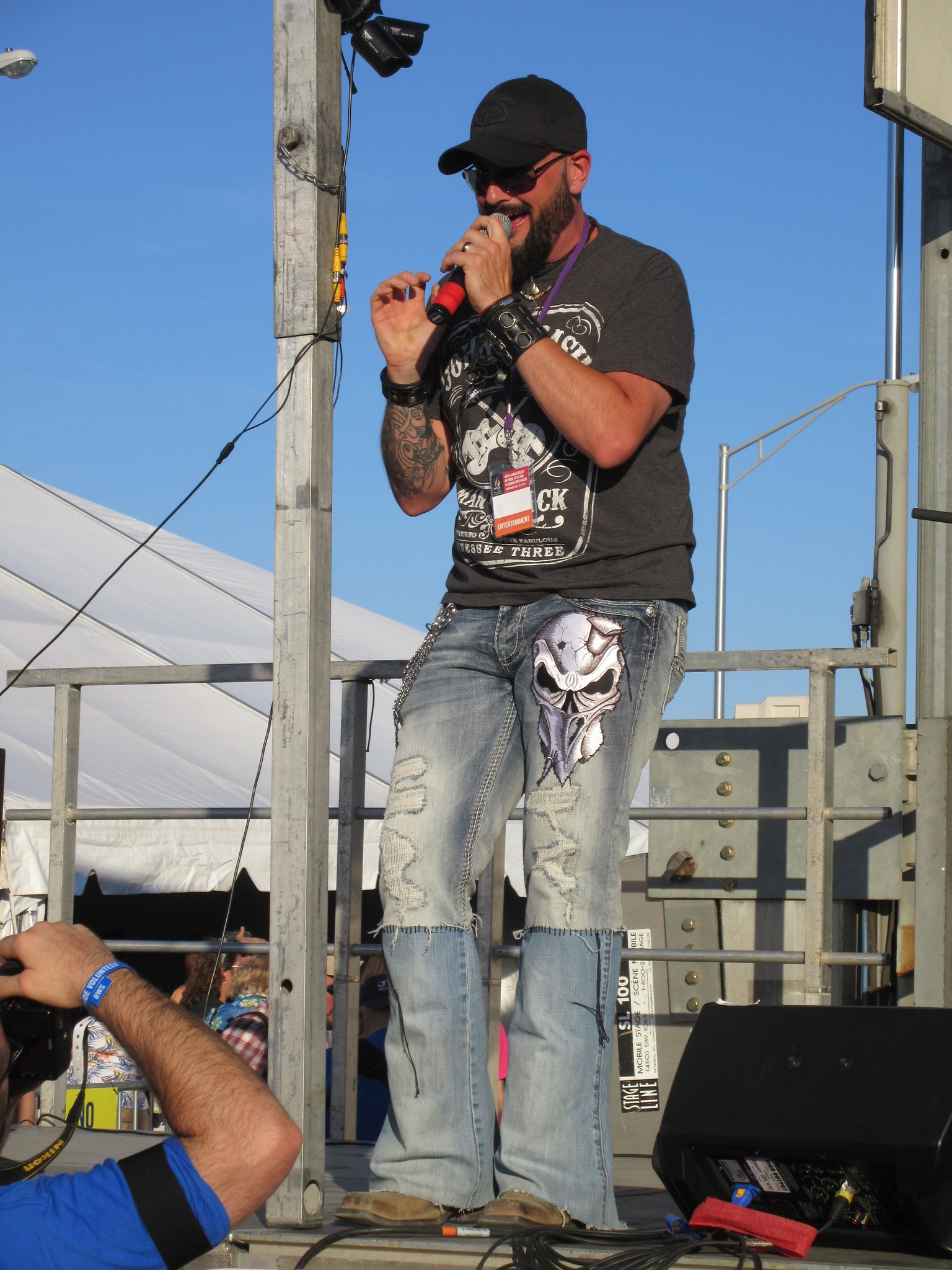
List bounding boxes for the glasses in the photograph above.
[327,984,335,994]
[462,152,570,195]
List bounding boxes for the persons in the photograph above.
[163,924,509,1144]
[0,917,301,1270]
[358,72,704,1241]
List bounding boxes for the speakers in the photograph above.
[651,997,951,1255]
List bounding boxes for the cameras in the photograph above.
[1,957,89,1106]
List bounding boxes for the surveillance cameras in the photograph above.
[322,0,430,78]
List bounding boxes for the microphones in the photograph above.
[426,212,512,325]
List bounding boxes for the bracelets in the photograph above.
[475,294,519,331]
[82,960,137,1012]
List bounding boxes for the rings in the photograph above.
[461,241,472,253]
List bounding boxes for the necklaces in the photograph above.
[514,277,554,301]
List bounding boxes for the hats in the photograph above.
[360,974,390,1010]
[438,75,588,175]
[327,955,335,976]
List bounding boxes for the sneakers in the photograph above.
[336,1191,485,1227]
[476,1190,577,1230]
